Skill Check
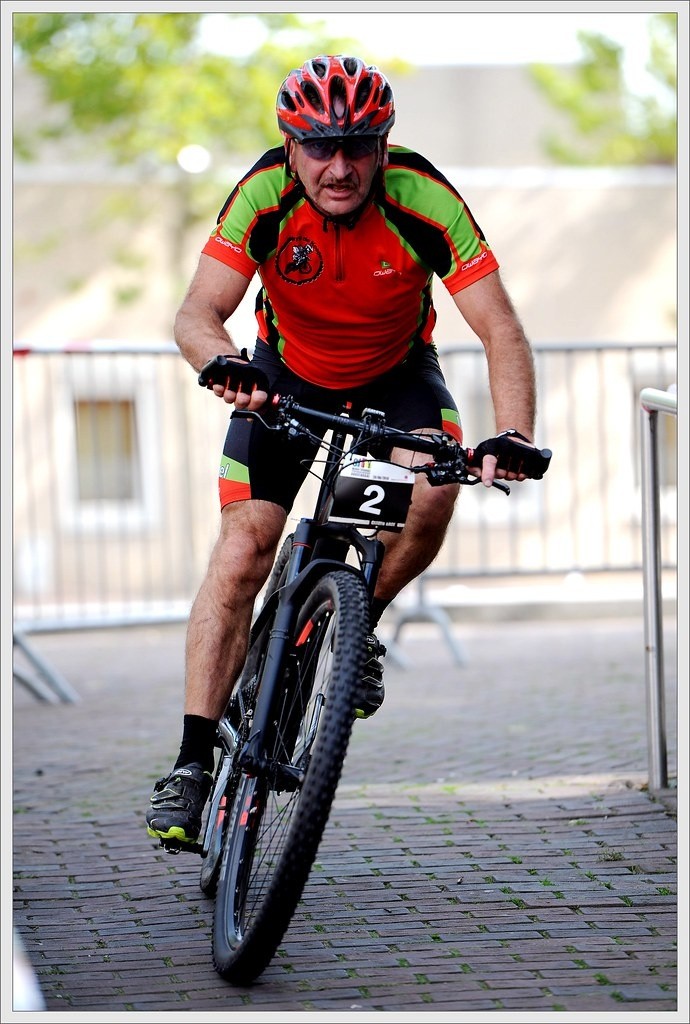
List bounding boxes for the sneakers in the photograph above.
[145,764,214,839]
[355,619,386,717]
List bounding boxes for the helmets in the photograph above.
[276,54,395,137]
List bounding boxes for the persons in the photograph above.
[145,46,539,844]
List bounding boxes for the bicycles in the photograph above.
[156,355,553,991]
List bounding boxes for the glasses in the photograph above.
[292,134,381,161]
